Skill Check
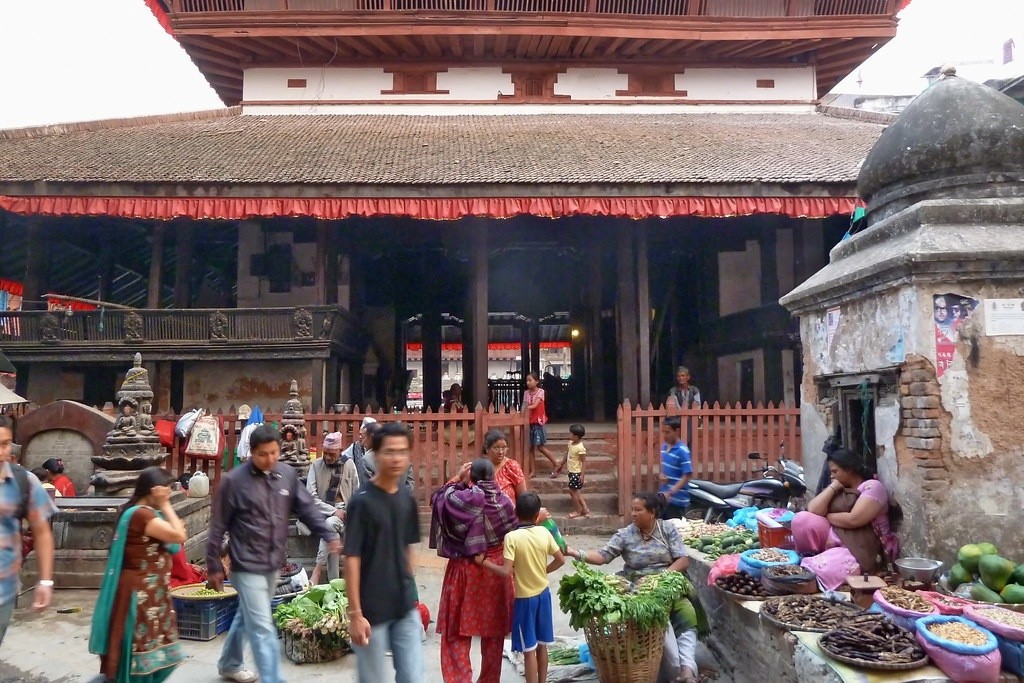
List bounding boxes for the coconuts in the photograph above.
[948,541,1024,604]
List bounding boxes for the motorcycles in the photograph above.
[685,440,807,524]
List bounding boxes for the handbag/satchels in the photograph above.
[175,408,202,438]
[155,419,176,448]
[181,416,225,459]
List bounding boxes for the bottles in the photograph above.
[188,472,209,498]
[393,406,398,414]
[541,518,568,556]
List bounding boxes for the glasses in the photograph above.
[490,447,509,452]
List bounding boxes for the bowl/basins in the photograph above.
[895,557,943,583]
[334,404,352,413]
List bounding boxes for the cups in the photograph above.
[175,482,181,490]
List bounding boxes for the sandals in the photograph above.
[529,473,536,479]
[550,463,562,477]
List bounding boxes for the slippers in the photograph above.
[674,677,698,683]
[565,512,581,518]
[580,511,591,518]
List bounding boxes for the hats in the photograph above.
[323,431,342,449]
[360,417,376,429]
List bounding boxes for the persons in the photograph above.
[343,421,427,683]
[170,542,206,590]
[556,424,590,519]
[658,418,693,518]
[521,371,562,478]
[664,365,702,445]
[295,417,415,584]
[468,431,527,508]
[89,467,188,683]
[437,385,468,425]
[474,492,565,683]
[560,492,709,683]
[430,458,519,683]
[206,425,341,683]
[791,449,900,591]
[0,415,76,646]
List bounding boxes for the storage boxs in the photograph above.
[170,580,239,641]
[757,519,795,552]
[271,598,286,639]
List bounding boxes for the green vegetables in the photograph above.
[548,647,581,665]
[557,558,691,633]
[272,577,351,639]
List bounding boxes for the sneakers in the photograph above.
[218,668,257,682]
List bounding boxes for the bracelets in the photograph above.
[828,485,837,494]
[38,579,55,586]
[481,557,486,564]
[348,609,362,615]
[575,550,586,562]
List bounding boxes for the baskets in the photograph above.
[759,522,795,549]
[170,584,238,599]
[713,575,768,601]
[582,611,665,683]
[760,594,865,632]
[816,630,931,669]
